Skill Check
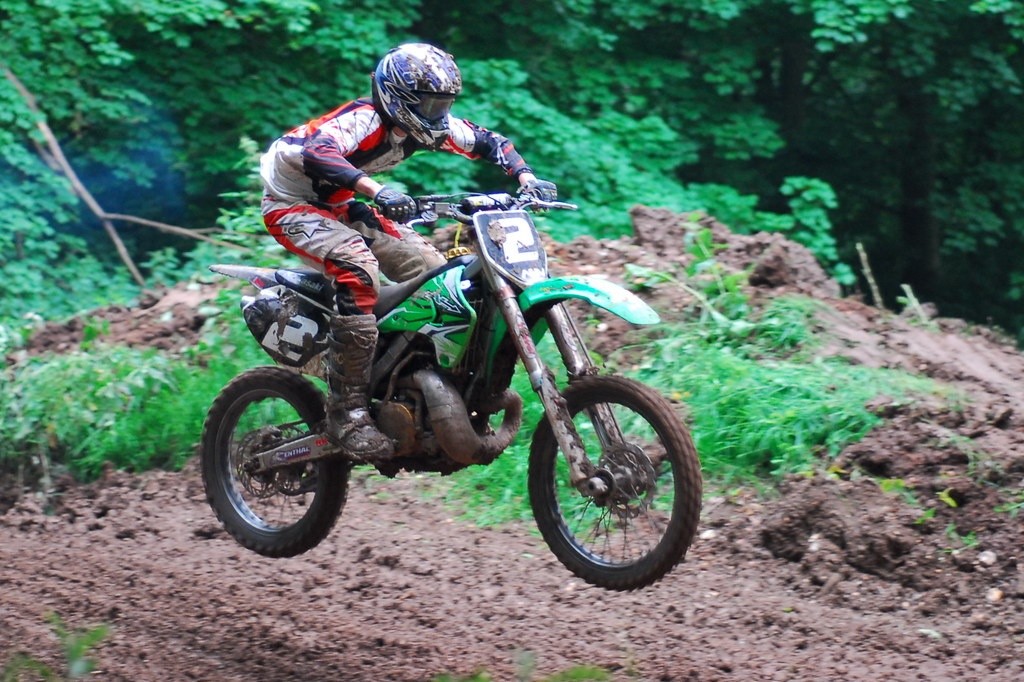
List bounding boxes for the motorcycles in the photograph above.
[200,190,703,592]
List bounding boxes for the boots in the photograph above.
[325,314,393,461]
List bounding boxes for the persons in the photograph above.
[258,42,558,461]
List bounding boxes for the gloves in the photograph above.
[374,186,418,225]
[517,177,558,215]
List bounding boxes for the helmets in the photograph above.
[376,43,462,149]
